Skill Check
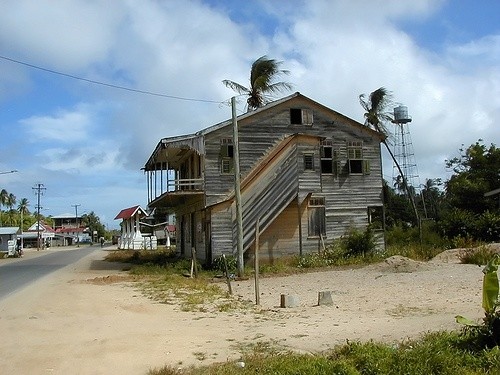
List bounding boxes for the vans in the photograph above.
[112,235,121,245]
